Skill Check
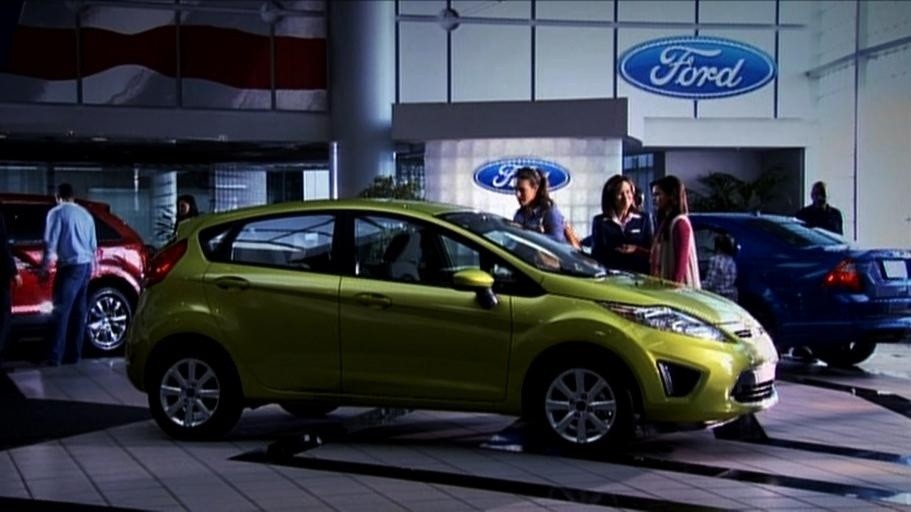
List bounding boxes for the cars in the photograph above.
[578,211,911,370]
[127,197,778,455]
[6,196,150,354]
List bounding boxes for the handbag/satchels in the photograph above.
[534,224,580,270]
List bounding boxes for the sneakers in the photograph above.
[64,354,80,364]
[45,356,61,368]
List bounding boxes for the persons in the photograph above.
[649,176,701,291]
[174,195,199,236]
[701,235,739,305]
[37,183,98,367]
[514,166,569,246]
[592,175,652,276]
[795,182,843,236]
[0,211,37,373]
[634,190,646,209]
